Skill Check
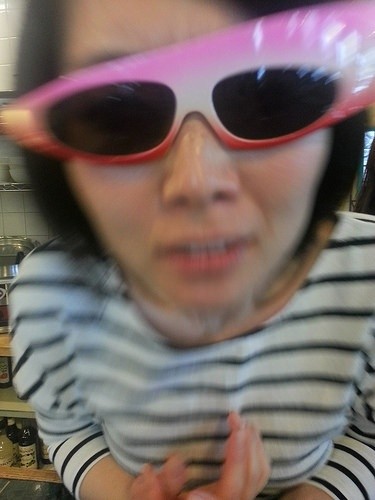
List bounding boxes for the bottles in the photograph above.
[0,417,52,470]
[0,356,12,389]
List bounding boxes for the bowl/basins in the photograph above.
[0,157,29,184]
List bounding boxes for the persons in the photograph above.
[0,0,374,500]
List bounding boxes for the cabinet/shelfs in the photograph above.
[0,278,64,487]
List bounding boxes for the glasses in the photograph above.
[1,1,375,167]
